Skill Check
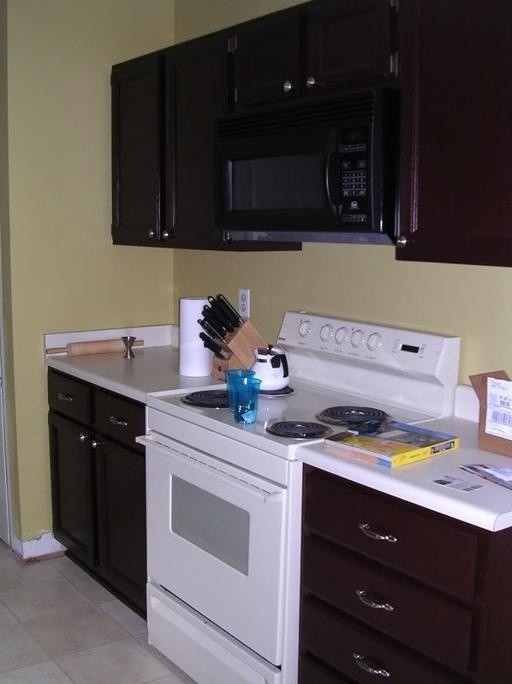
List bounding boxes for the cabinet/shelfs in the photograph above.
[229,1,401,105]
[298,472,510,684]
[393,0,512,267]
[111,35,303,253]
[45,368,148,622]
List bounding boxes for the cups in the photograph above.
[122,336,137,358]
[225,368,262,424]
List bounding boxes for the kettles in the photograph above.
[249,348,291,390]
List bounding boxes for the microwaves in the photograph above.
[206,80,388,234]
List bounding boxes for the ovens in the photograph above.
[136,396,302,678]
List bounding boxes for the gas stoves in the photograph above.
[181,375,384,442]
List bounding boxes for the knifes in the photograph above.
[196,292,246,360]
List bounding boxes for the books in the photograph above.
[324,415,458,468]
